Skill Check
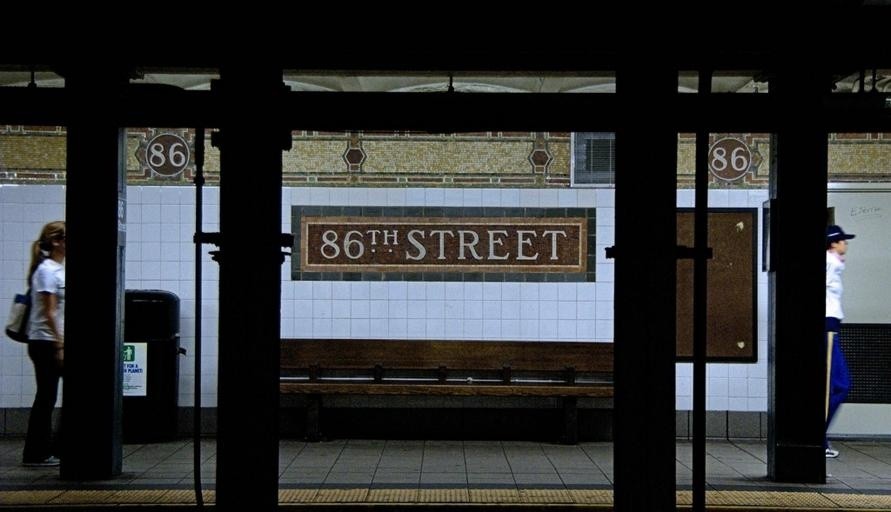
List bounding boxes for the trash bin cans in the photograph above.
[123,290,180,444]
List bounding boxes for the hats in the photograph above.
[826,223,855,242]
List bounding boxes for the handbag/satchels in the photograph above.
[5,293,31,345]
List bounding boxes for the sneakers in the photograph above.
[827,449,839,459]
[22,456,60,468]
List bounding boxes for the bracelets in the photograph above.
[56,346,65,352]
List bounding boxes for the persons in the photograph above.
[21,220,67,468]
[824,226,857,458]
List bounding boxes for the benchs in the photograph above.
[279,337,614,445]
